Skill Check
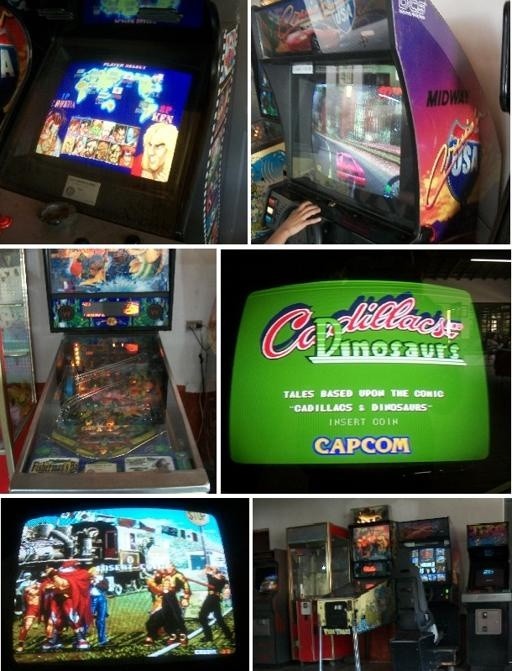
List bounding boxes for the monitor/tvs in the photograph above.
[473,563,505,588]
[408,546,447,584]
[310,82,401,198]
[259,575,277,592]
[10,507,237,663]
[226,279,490,465]
[34,54,192,191]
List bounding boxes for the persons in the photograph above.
[266,202,322,243]
